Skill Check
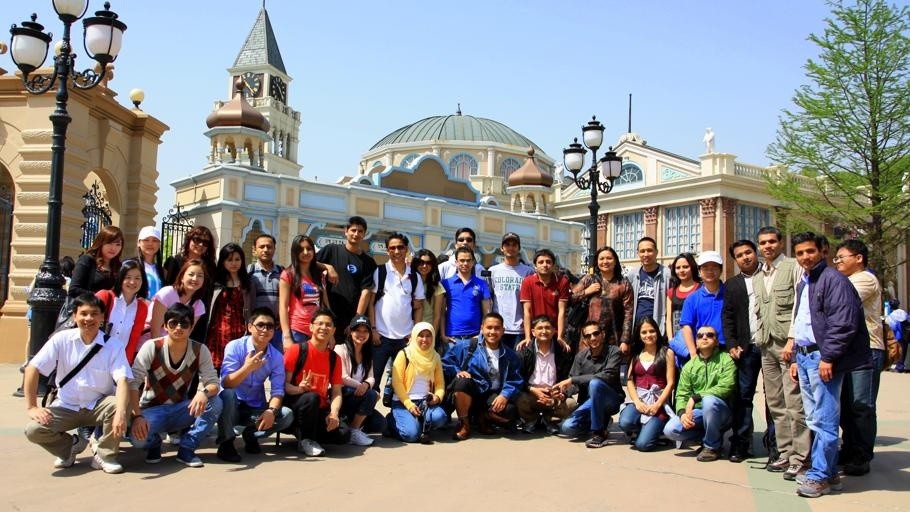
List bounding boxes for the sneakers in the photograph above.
[243,428,260,454]
[145,439,162,464]
[474,414,559,436]
[298,439,325,457]
[167,432,203,467]
[350,429,376,446]
[454,421,470,440]
[729,434,875,498]
[585,417,668,449]
[217,448,242,462]
[54,427,124,473]
[697,448,719,461]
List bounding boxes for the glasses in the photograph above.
[313,321,334,328]
[419,260,431,266]
[696,332,716,338]
[251,322,274,330]
[584,330,600,339]
[389,245,405,251]
[169,320,191,329]
[457,238,473,242]
[192,237,210,247]
[832,254,856,263]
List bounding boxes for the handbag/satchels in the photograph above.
[668,331,690,358]
[567,299,588,328]
[445,338,478,408]
[53,290,113,340]
[382,349,409,408]
[879,320,903,370]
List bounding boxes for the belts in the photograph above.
[796,345,818,355]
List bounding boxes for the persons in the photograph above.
[788,232,885,498]
[884,297,910,373]
[24,216,812,480]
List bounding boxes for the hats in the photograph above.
[502,232,520,246]
[138,226,161,241]
[350,315,370,329]
[698,251,723,266]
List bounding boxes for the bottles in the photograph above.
[383,378,394,406]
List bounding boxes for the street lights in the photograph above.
[10,0,126,399]
[563,113,622,272]
